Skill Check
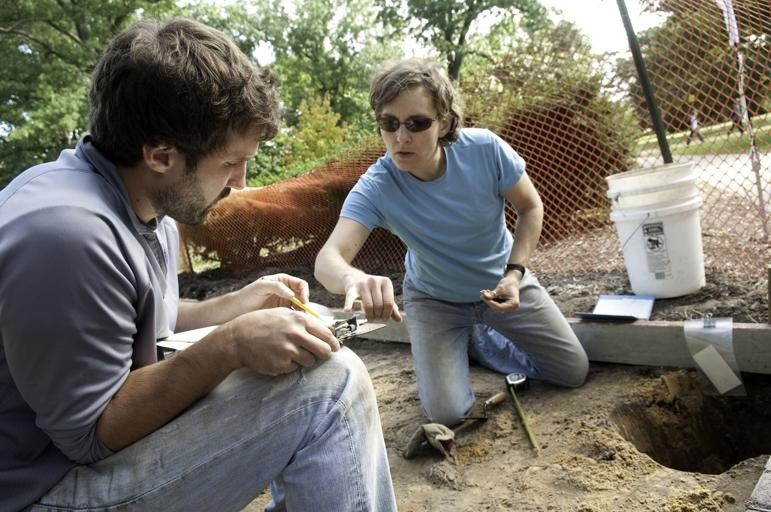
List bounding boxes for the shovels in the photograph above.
[458,392,506,419]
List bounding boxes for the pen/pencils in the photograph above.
[291,298,321,320]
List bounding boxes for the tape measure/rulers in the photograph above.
[506,372,539,448]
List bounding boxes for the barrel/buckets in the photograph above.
[605,161,706,299]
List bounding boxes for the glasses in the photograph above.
[376,115,440,132]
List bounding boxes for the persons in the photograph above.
[0,17,398,512]
[727,98,745,136]
[314,56,590,428]
[685,110,705,148]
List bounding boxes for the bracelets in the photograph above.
[504,262,524,279]
